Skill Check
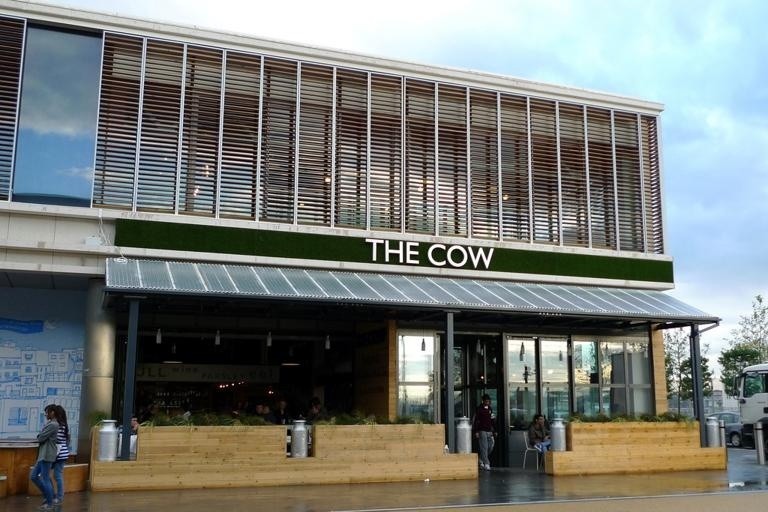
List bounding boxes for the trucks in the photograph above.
[733,364,768,448]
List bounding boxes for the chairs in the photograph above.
[521,431,542,470]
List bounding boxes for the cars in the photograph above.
[689,410,742,447]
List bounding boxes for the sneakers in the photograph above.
[534,444,543,452]
[481,464,491,470]
[40,499,61,510]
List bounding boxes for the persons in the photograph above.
[117,398,321,459]
[52,406,70,506]
[474,394,498,470]
[30,404,59,511]
[528,414,552,454]
